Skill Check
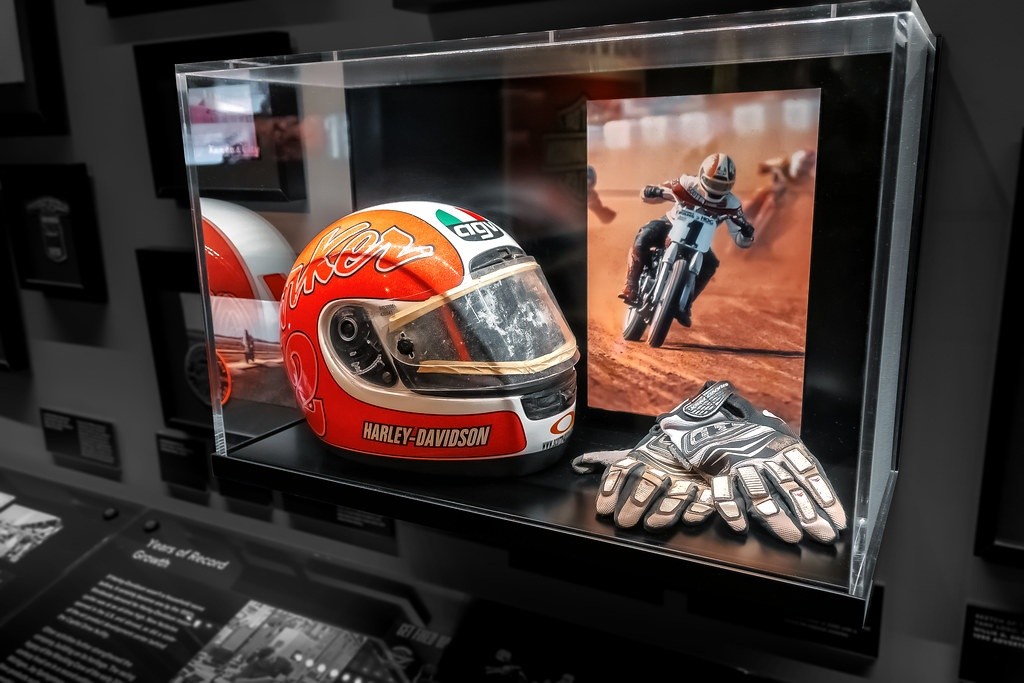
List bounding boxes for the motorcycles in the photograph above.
[726,165,812,263]
[622,186,757,347]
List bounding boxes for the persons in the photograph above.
[618,150,754,327]
[739,147,818,248]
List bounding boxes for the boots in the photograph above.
[673,275,713,327]
[618,247,642,301]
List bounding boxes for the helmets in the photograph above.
[587,166,597,189]
[789,149,815,178]
[279,201,580,460]
[699,153,736,203]
[200,197,297,369]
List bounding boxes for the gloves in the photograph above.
[572,381,851,544]
[740,222,756,238]
[644,186,664,203]
